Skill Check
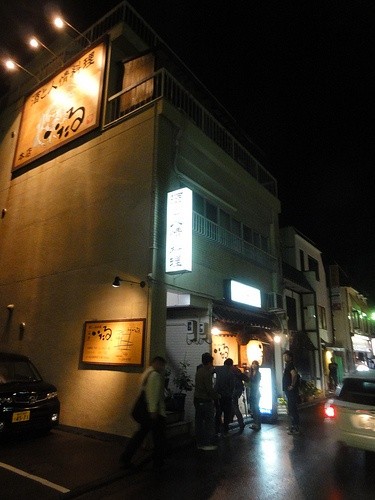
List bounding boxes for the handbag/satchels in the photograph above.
[126,388,167,433]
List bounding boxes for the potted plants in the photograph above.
[158,349,193,421]
[300,379,318,402]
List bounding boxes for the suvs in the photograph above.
[0,353,60,444]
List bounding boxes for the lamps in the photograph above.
[112,277,146,289]
[3,13,92,83]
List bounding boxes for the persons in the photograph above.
[329,358,338,392]
[119,356,169,468]
[194,353,216,451]
[213,359,249,436]
[282,351,300,434]
[246,361,261,431]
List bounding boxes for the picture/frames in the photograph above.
[10,33,109,174]
[79,318,147,368]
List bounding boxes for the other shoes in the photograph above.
[286,424,301,435]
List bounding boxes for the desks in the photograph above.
[165,419,193,434]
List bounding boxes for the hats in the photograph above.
[201,352,214,364]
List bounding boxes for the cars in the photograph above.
[325,369,375,452]
